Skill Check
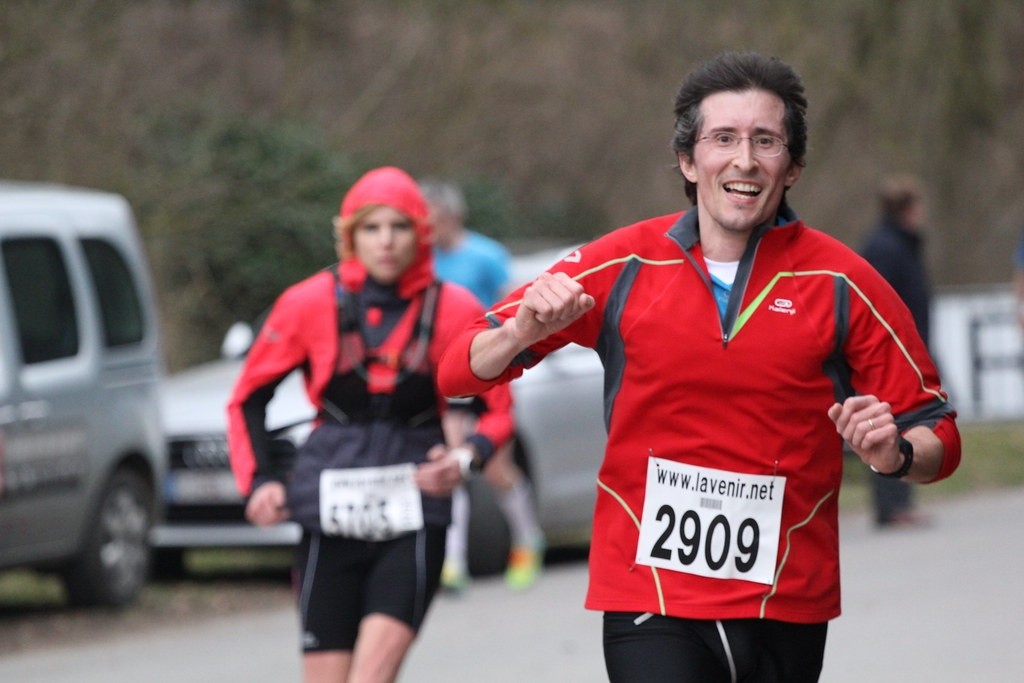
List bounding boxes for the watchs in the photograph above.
[450,448,480,479]
[870,435,914,478]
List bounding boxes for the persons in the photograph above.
[227,167,514,683]
[439,52,961,683]
[420,172,544,591]
[858,178,937,526]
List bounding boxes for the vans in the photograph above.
[1,178,167,614]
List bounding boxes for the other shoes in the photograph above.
[439,563,472,592]
[501,544,546,591]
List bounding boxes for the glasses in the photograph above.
[694,132,788,158]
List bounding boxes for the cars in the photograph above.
[151,243,608,584]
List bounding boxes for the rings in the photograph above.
[869,419,875,430]
